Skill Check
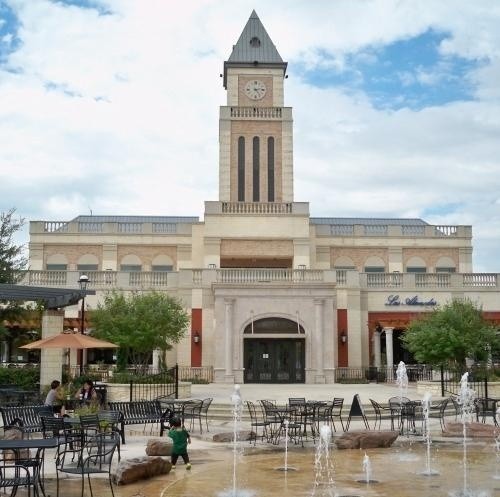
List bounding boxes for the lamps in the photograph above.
[340,330,348,345]
[192,329,200,344]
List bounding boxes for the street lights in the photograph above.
[78,274,89,376]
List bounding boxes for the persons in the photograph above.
[74,380,97,405]
[167,416,192,469]
[44,379,66,414]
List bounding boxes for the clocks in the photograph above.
[244,78,267,100]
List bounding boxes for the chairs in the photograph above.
[246,394,500,447]
[1,387,215,497]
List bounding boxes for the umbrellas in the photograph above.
[18,327,120,381]
